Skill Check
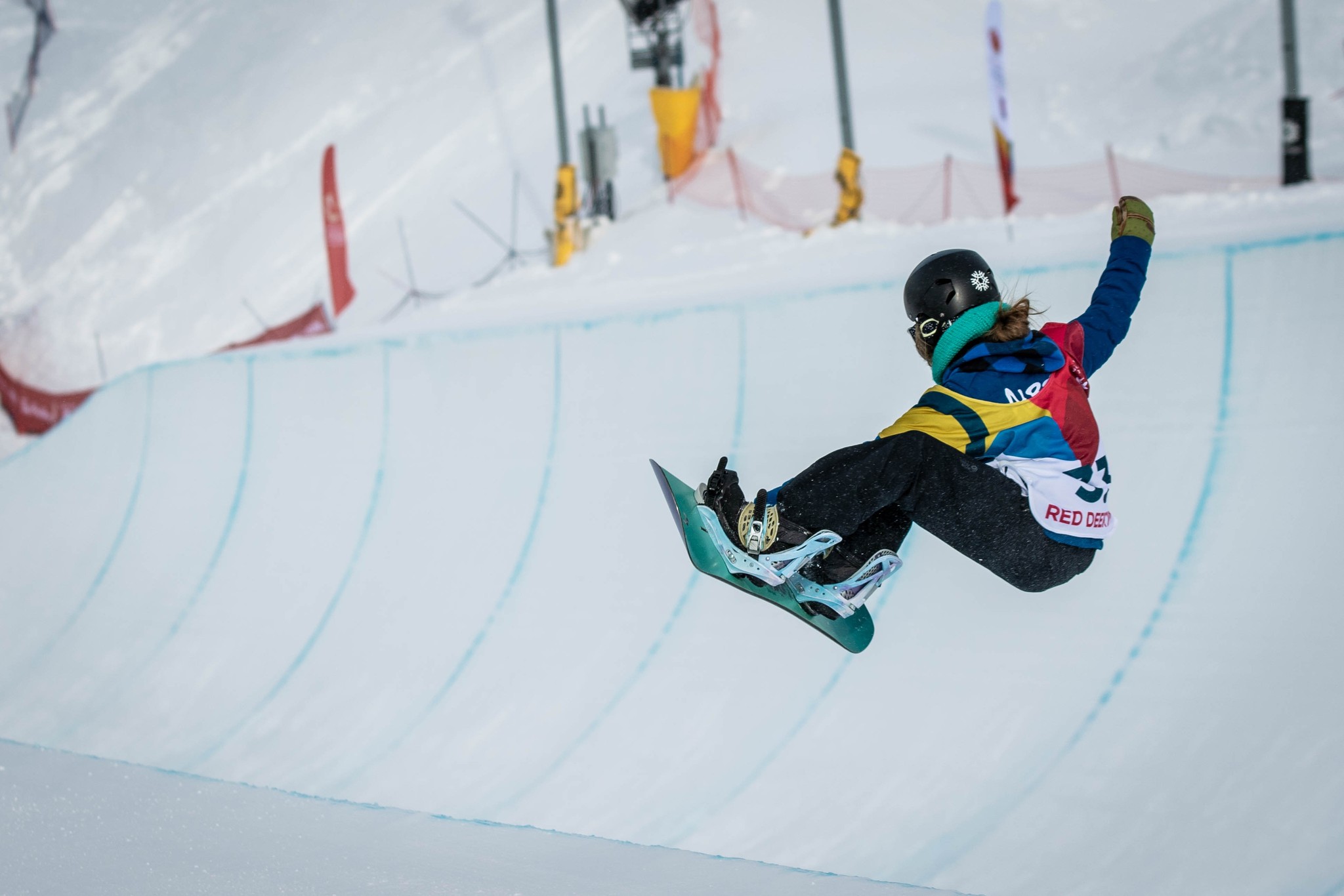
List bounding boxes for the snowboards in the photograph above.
[649,457,875,654]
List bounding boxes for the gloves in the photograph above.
[1111,196,1156,251]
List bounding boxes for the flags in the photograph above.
[324,147,355,312]
[983,0,1017,214]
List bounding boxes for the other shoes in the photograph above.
[797,561,856,618]
[709,470,760,550]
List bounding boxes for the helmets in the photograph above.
[902,249,1002,358]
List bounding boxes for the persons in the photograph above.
[706,196,1155,622]
